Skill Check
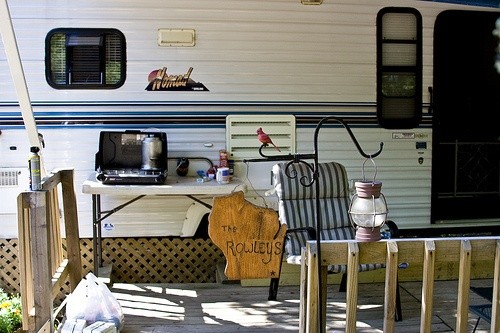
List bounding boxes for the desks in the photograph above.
[82,181,248,278]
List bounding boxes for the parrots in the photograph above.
[257,127,281,153]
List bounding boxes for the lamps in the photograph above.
[348,154,389,242]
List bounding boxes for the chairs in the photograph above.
[267,161,409,323]
